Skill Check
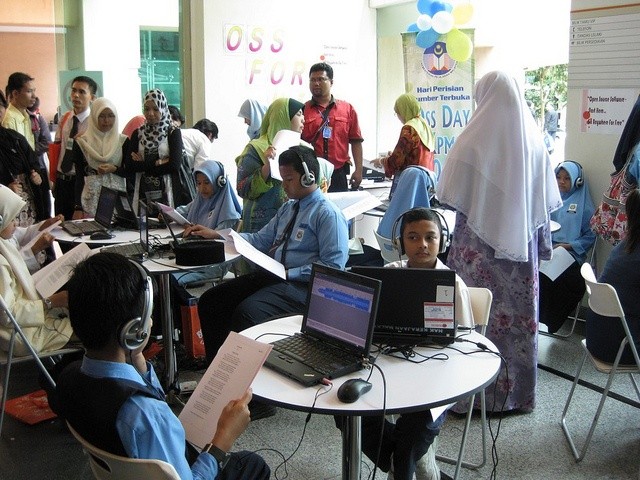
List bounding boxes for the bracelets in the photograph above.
[159,159,163,166]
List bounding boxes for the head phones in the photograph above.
[562,161,586,192]
[289,146,316,189]
[392,207,450,256]
[214,161,227,188]
[120,257,155,351]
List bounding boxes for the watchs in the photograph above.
[43,296,53,311]
[201,442,231,474]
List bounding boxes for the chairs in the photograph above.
[183,219,244,290]
[538,235,598,339]
[560,261,639,461]
[373,229,409,267]
[64,418,182,480]
[435,285,494,480]
[355,176,400,225]
[0,293,85,438]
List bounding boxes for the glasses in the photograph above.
[311,78,328,82]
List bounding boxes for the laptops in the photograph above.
[100,199,148,259]
[350,267,455,344]
[111,189,165,228]
[263,262,383,387]
[61,185,118,236]
[159,208,217,246]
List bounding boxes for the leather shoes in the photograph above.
[249,402,278,420]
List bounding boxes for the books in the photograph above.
[178,330,276,454]
[215,228,287,281]
[154,201,190,225]
[539,246,576,281]
[30,241,94,299]
[267,129,314,181]
[21,220,62,250]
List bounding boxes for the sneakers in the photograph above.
[387,452,395,480]
[415,436,441,480]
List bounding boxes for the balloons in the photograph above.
[429,1,447,17]
[415,27,441,48]
[446,29,473,63]
[406,23,420,32]
[417,13,432,32]
[431,11,454,34]
[452,5,475,25]
[416,0,432,14]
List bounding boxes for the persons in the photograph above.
[543,101,558,152]
[300,62,363,192]
[330,207,474,480]
[159,159,241,287]
[236,98,305,272]
[1,72,36,152]
[613,96,640,245]
[179,119,218,171]
[51,74,98,222]
[122,104,185,136]
[122,89,182,210]
[376,165,437,265]
[526,100,538,124]
[27,95,53,220]
[0,91,43,227]
[0,185,85,357]
[368,93,433,173]
[539,160,600,334]
[587,189,640,364]
[238,99,270,139]
[438,72,562,413]
[70,97,134,219]
[197,145,349,366]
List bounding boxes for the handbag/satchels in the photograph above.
[588,147,638,247]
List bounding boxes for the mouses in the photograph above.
[337,378,372,403]
[90,230,114,241]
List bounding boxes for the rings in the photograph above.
[137,159,139,161]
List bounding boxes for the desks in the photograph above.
[322,189,384,240]
[235,313,502,480]
[45,213,187,246]
[85,237,243,409]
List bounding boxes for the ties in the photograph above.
[267,202,299,264]
[60,116,79,173]
